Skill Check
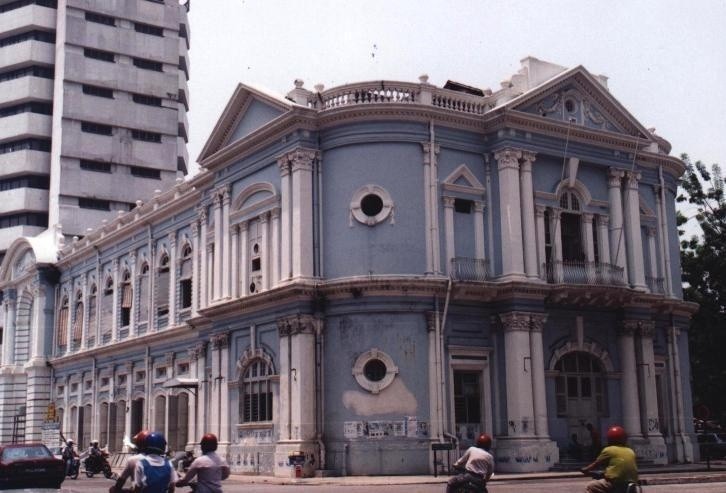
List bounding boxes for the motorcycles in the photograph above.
[64,456,79,478]
[80,452,110,477]
[578,467,642,492]
[454,464,494,492]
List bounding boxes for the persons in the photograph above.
[134,432,179,493]
[63,438,79,463]
[89,441,102,467]
[110,430,154,493]
[175,434,229,493]
[88,442,94,458]
[582,425,638,493]
[586,421,600,455]
[570,434,585,458]
[446,434,495,493]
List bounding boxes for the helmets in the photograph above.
[132,430,150,449]
[477,435,492,450]
[201,434,217,451]
[66,439,73,446]
[92,440,98,445]
[607,427,627,445]
[145,432,166,455]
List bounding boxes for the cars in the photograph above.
[0,442,65,486]
